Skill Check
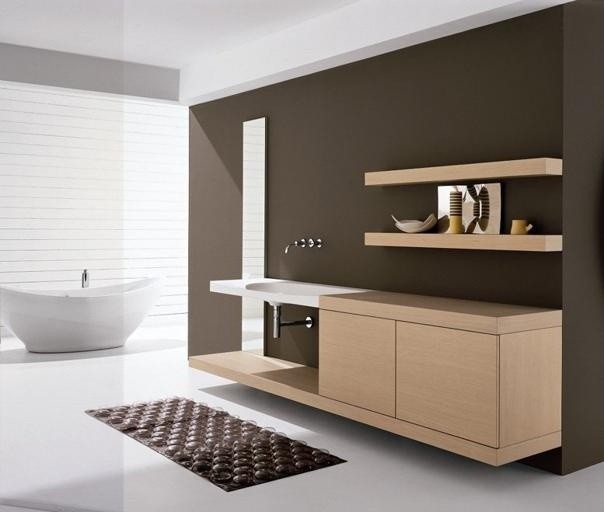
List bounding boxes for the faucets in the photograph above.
[82,268,89,288]
[284,237,307,255]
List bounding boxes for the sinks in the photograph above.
[248,277,353,296]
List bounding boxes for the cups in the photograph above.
[510,219,533,234]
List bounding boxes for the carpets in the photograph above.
[83,395,349,493]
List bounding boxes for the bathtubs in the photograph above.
[0,276,154,354]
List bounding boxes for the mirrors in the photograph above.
[242,116,268,356]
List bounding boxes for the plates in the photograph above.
[391,214,437,233]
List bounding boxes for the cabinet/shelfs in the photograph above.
[364,157,563,252]
[319,290,562,467]
